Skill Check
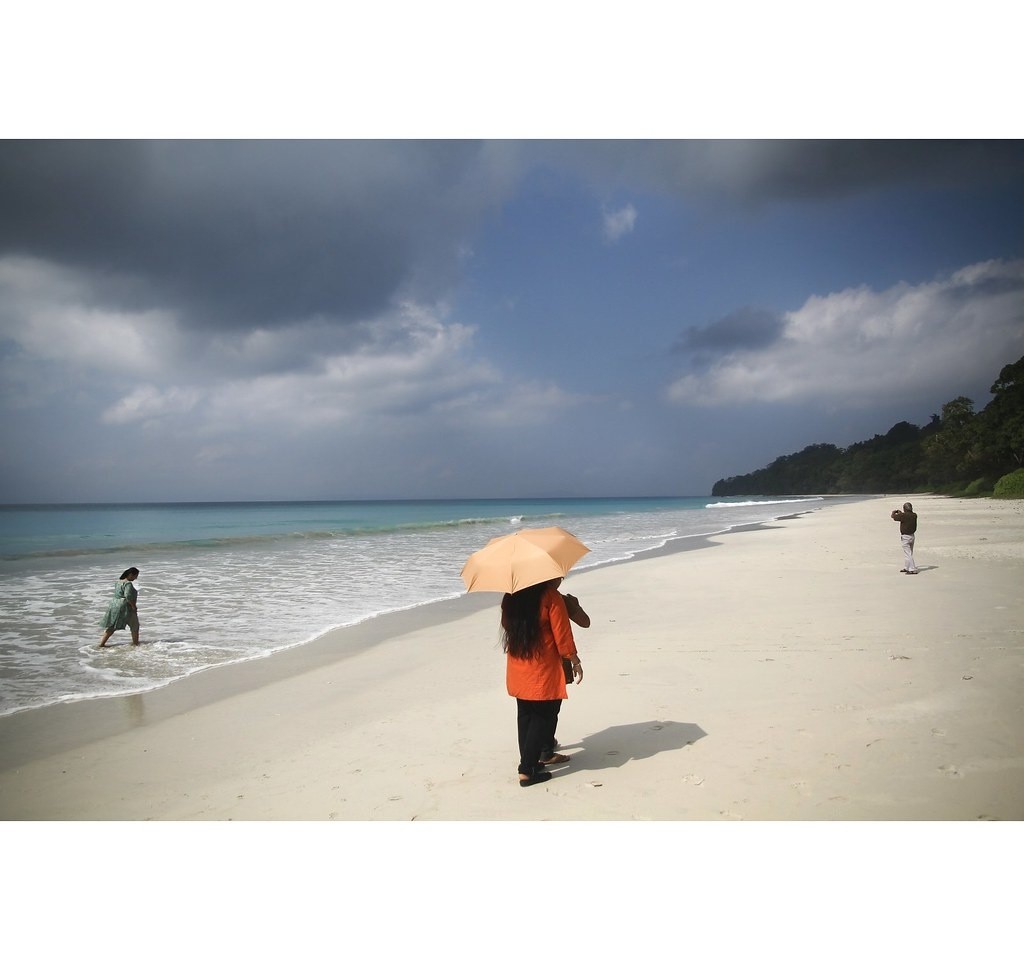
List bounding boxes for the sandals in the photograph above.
[535,761,545,771]
[905,571,917,574]
[900,569,908,572]
[540,753,570,763]
[519,770,552,786]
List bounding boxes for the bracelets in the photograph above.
[573,660,581,666]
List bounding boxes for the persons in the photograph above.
[891,502,918,574]
[501,577,590,787]
[100,567,140,646]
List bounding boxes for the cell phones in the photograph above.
[895,511,898,513]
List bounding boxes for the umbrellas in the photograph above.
[459,526,592,595]
